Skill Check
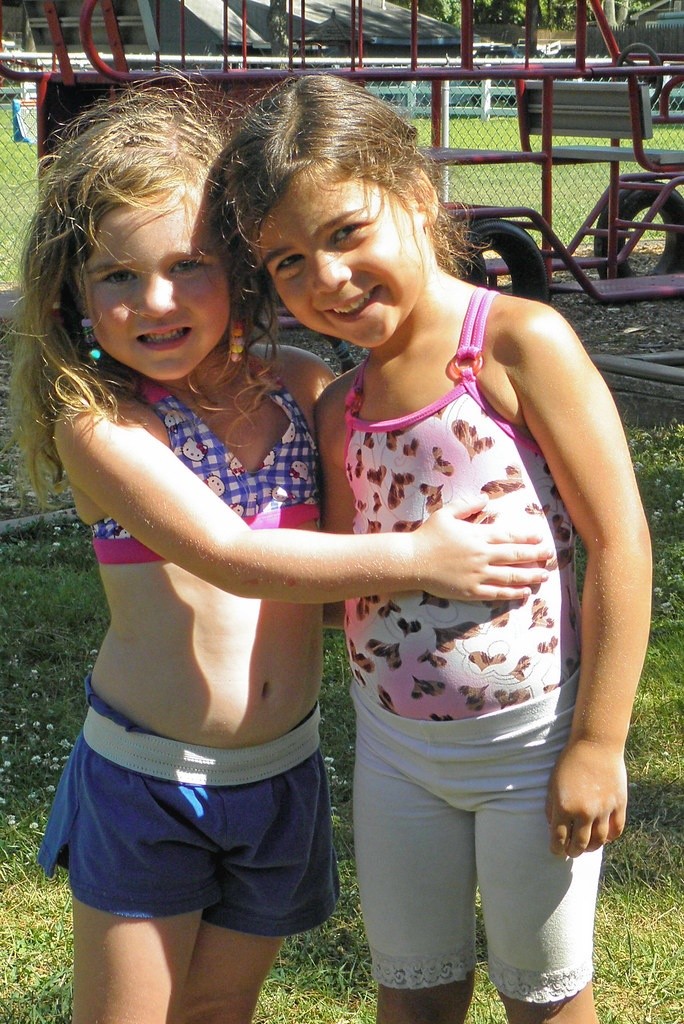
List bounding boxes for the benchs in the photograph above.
[515,78,684,173]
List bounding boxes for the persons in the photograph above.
[9,88,555,1024]
[222,73,653,1024]
